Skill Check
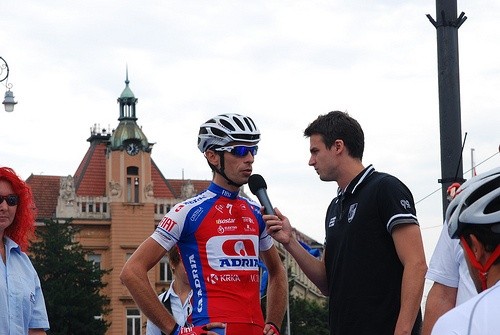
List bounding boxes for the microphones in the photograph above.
[248,173,280,232]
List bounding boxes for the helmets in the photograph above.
[446,167,500,240]
[198,113,260,153]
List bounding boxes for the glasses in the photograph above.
[0,194,18,207]
[212,145,259,157]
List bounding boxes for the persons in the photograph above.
[145,245,193,335]
[0,167,50,335]
[431,167,500,335]
[120,113,288,335]
[260,111,433,335]
[422,217,480,335]
